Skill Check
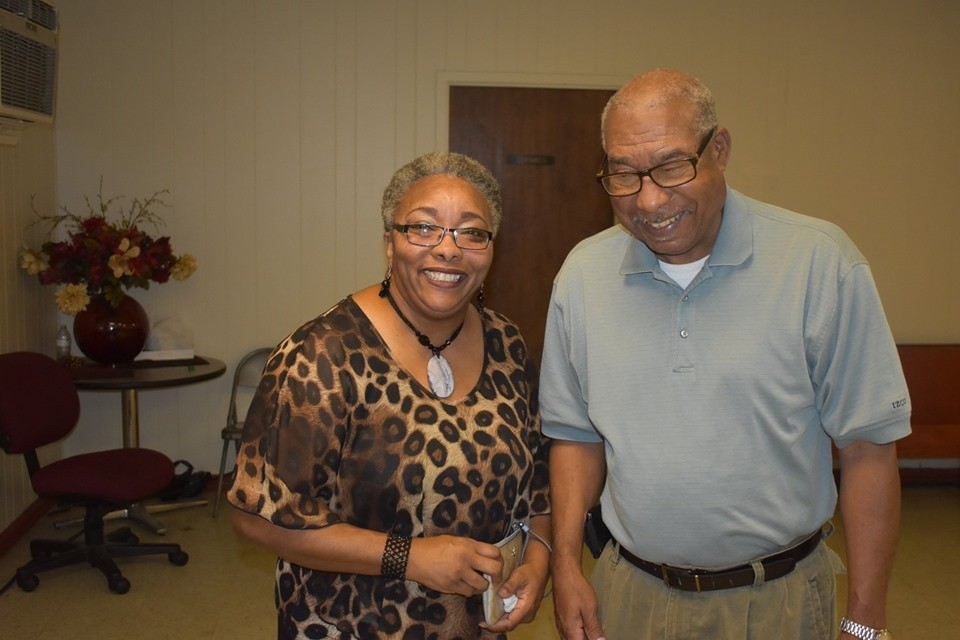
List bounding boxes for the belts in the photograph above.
[610,526,823,592]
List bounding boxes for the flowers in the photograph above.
[15,174,197,316]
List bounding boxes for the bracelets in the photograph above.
[380,531,412,582]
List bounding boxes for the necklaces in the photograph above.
[379,279,466,399]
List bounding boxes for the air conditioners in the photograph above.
[0,0,58,126]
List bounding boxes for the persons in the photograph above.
[535,70,909,640]
[222,151,554,640]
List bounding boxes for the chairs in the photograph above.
[214,346,275,518]
[0,352,189,594]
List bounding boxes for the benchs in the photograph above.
[832,344,960,484]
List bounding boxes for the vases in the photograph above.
[74,281,149,369]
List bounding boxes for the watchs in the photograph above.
[839,617,893,640]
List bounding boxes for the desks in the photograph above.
[55,356,226,535]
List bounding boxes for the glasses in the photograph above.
[595,122,717,197]
[384,223,492,250]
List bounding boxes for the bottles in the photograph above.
[57,324,72,358]
